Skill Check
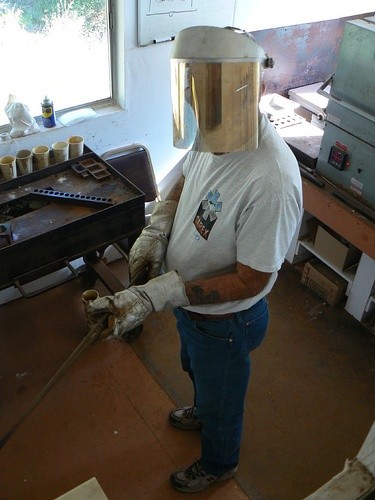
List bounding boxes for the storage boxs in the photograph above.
[299,258,347,307]
[312,223,362,271]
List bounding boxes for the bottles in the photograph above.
[80,290,104,329]
[40,96,57,129]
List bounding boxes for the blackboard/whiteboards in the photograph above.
[137,0,236,47]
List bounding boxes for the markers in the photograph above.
[154,36,175,44]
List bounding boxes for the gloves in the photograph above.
[87,200,189,344]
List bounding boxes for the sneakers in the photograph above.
[169,404,204,431]
[170,455,239,494]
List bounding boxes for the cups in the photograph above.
[51,141,69,163]
[0,155,16,180]
[32,145,49,169]
[67,135,84,157]
[16,149,33,175]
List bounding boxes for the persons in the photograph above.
[89,26,302,491]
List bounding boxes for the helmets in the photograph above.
[168,26,276,152]
